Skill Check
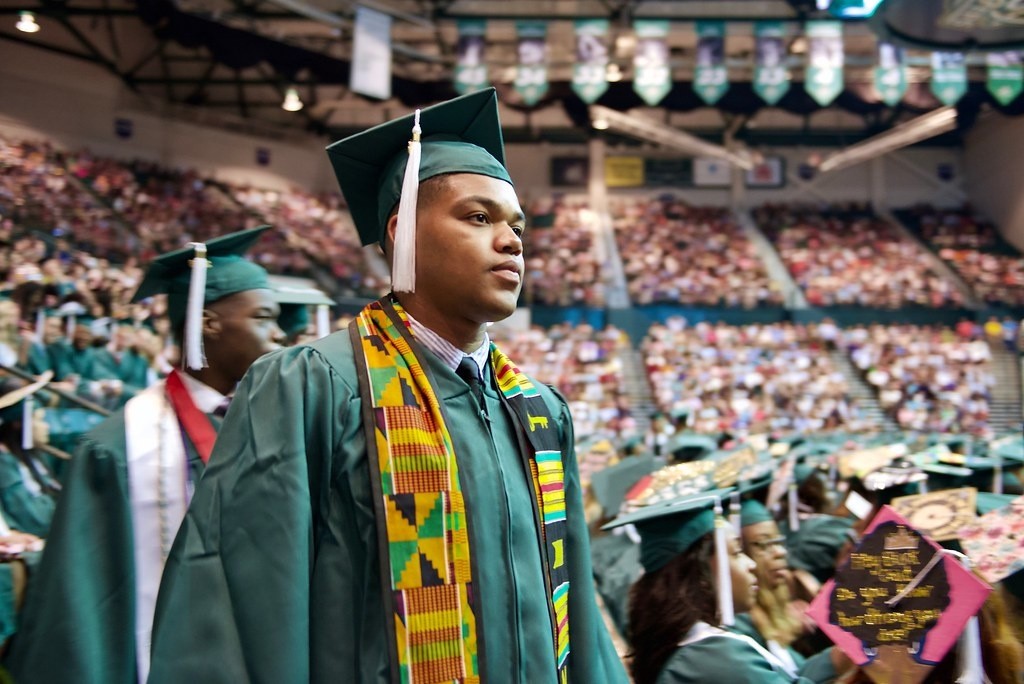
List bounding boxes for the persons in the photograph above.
[147,86,596,684]
[0,138,1024,683]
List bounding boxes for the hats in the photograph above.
[0,382,48,449]
[324,87,514,293]
[130,225,276,371]
[599,428,1024,631]
[0,299,165,348]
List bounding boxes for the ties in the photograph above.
[458,357,485,415]
